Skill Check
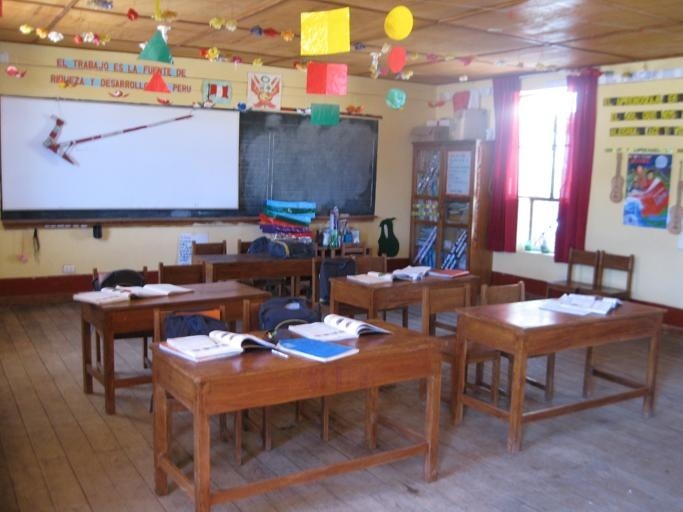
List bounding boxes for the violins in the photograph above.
[610,152,623,203]
[669,179,682,235]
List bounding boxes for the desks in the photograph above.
[450,295,667,453]
[148,318,448,512]
[73,280,272,415]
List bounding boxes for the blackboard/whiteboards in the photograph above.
[0,98,377,229]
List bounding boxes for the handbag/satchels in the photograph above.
[163,314,225,339]
[267,240,313,259]
[320,257,355,300]
[260,297,318,332]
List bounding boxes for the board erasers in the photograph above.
[169,210,192,218]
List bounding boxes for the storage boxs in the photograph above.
[449,108,487,141]
[411,126,449,142]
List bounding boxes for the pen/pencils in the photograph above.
[271,350,289,358]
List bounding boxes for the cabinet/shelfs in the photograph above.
[410,139,495,296]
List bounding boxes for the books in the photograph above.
[346,265,470,285]
[72,282,193,305]
[539,293,623,316]
[158,313,392,366]
